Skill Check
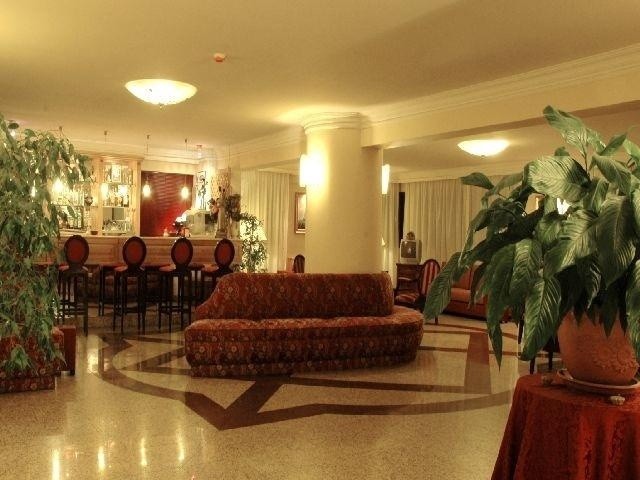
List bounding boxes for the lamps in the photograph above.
[124,79,197,106]
[455,137,511,158]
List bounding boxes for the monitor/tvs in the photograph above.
[400,238,422,264]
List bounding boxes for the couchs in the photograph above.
[443,264,511,323]
[183,271,425,377]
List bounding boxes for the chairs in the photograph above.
[55,234,236,337]
[393,259,441,324]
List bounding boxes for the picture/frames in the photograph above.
[295,191,306,234]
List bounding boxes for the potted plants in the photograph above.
[422,104,640,393]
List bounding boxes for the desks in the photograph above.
[496,374,640,478]
